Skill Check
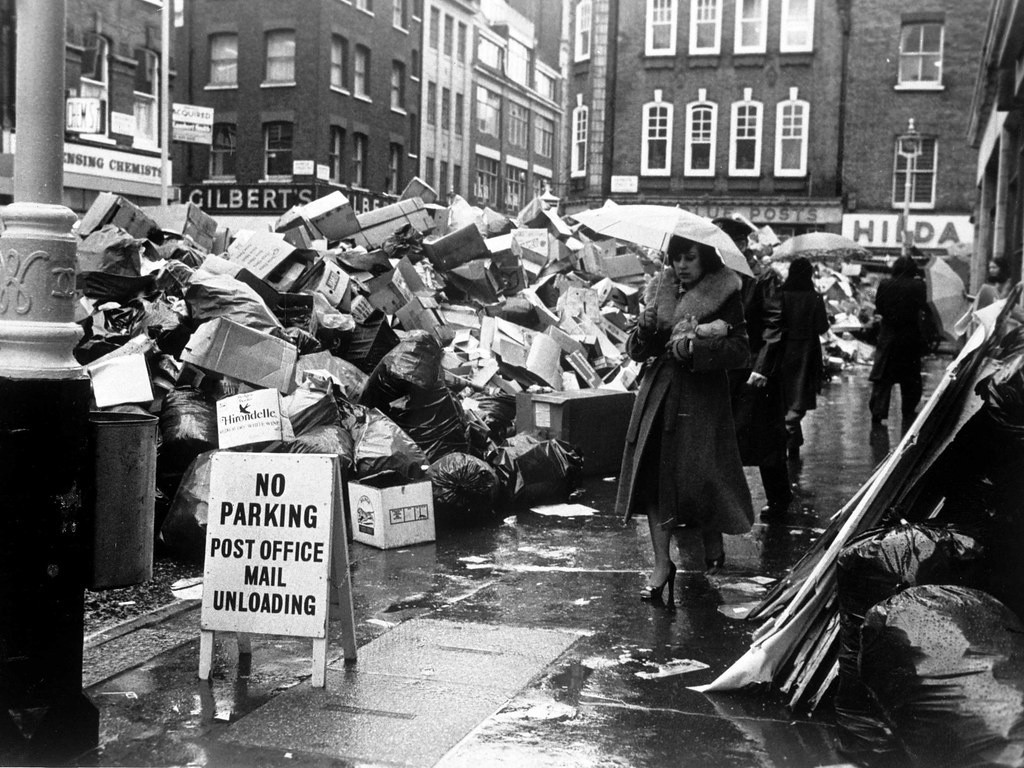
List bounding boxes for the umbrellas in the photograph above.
[769,230,873,292]
[569,203,757,308]
[923,252,968,344]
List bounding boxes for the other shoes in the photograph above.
[761,501,793,516]
[787,447,799,469]
[872,413,881,424]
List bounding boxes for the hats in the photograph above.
[713,217,753,241]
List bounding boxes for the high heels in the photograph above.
[702,531,724,575]
[641,561,677,601]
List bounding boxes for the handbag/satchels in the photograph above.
[917,280,934,343]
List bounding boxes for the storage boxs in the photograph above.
[74,177,655,550]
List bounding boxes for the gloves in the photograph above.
[637,305,657,338]
[664,336,692,366]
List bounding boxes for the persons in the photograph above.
[867,255,928,424]
[986,258,1013,299]
[613,234,756,600]
[781,257,832,461]
[709,217,794,523]
